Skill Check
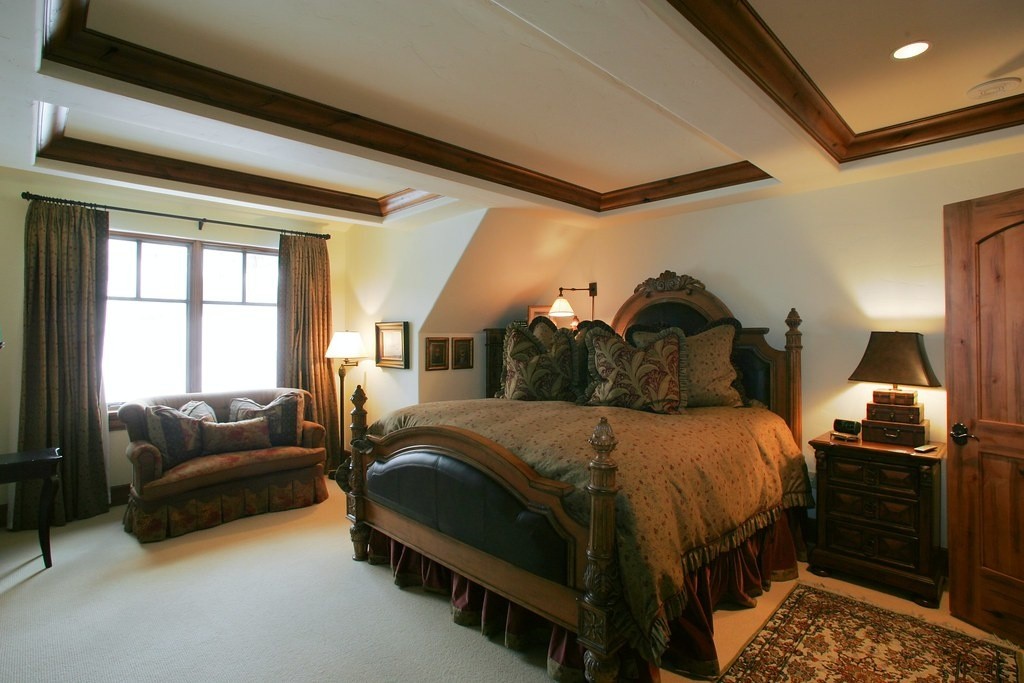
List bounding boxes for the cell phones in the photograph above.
[914,445,938,452]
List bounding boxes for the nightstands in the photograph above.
[807,430,948,610]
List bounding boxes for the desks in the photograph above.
[0,446,63,569]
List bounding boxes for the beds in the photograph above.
[334,270,813,683]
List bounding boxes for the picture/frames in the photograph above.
[375,321,409,369]
[452,337,474,369]
[426,337,449,371]
[527,304,558,326]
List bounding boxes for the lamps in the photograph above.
[325,330,368,478]
[548,283,597,321]
[571,316,579,328]
[847,331,942,390]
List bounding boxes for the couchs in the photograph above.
[117,387,330,544]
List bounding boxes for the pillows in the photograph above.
[576,327,690,416]
[146,399,218,471]
[228,391,304,445]
[625,318,752,408]
[495,324,581,402]
[528,316,591,353]
[200,415,272,456]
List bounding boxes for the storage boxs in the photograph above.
[862,417,931,446]
[866,401,924,423]
[872,389,918,405]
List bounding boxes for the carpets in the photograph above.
[713,579,1024,683]
